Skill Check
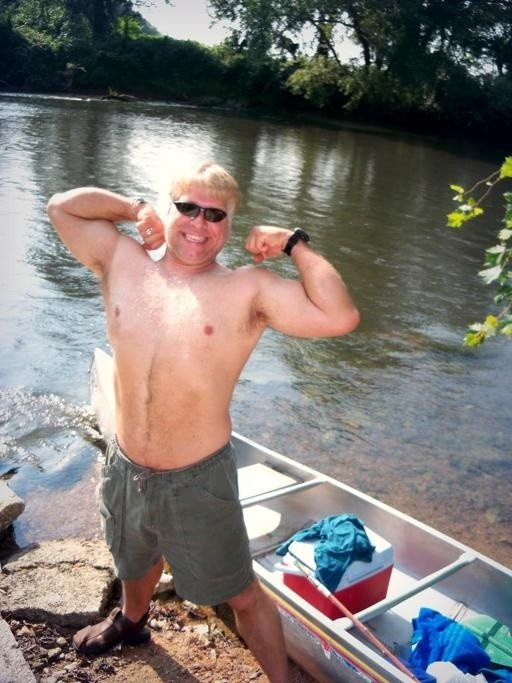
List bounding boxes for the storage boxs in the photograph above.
[283,522,396,621]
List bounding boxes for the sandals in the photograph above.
[73,609,154,654]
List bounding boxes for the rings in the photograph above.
[145,225,153,235]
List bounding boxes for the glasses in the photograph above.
[174,200,230,223]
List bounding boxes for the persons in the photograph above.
[44,163,359,683]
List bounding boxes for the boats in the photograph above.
[87,343,510,681]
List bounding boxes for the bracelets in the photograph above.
[128,196,146,219]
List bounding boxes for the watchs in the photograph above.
[281,225,312,255]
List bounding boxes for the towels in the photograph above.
[272,510,375,596]
[390,608,512,682]
